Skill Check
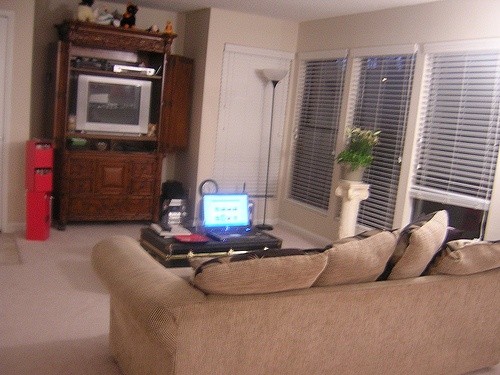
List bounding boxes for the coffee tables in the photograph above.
[140,227,284,266]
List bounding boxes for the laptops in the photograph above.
[201,194,260,241]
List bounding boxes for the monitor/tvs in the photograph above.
[75,74,152,137]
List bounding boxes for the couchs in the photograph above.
[90,235,500,375]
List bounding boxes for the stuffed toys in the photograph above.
[73,0,140,29]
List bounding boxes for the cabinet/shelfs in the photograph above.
[52,21,191,231]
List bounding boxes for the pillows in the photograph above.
[381,210,450,282]
[426,240,500,275]
[315,228,400,288]
[191,247,334,296]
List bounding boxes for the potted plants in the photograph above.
[333,125,382,181]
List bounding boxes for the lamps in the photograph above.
[256,67,288,230]
